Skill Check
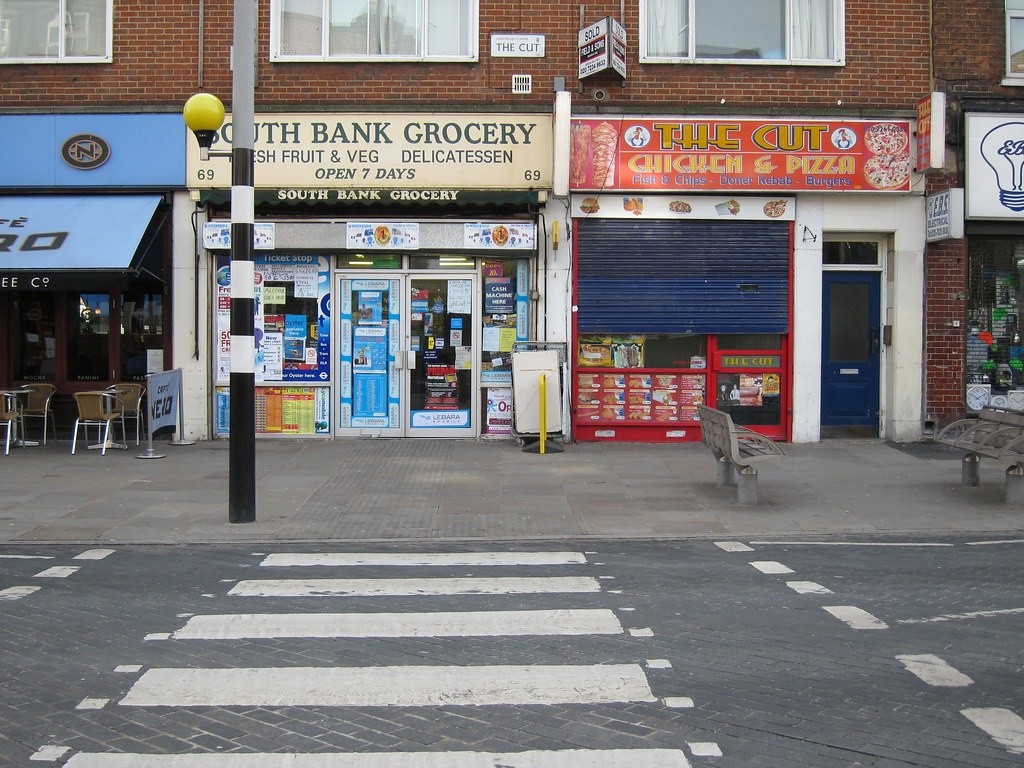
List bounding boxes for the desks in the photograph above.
[0,389,39,450]
[88,390,128,450]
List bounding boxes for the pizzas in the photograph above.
[658,378,672,386]
[579,346,611,366]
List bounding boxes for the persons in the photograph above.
[355,349,367,364]
[756,387,763,395]
[753,377,762,386]
[719,383,740,399]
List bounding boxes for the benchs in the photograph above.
[697,401,786,507]
[933,403,1024,506]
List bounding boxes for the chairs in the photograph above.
[13,383,57,445]
[70,392,127,456]
[0,393,26,455]
[106,384,148,446]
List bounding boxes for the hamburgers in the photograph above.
[579,376,593,388]
[578,392,592,404]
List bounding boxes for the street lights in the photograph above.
[182,91,256,525]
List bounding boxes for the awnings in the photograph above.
[962,112,1024,234]
[0,191,174,287]
